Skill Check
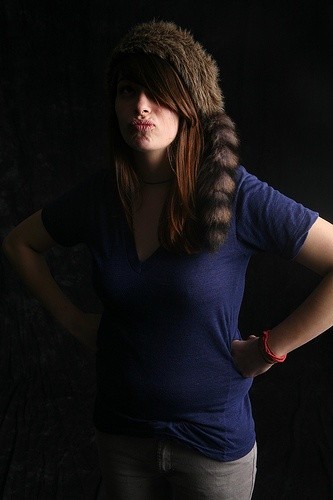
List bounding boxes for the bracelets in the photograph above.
[258,330,286,364]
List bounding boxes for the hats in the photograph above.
[104,23,238,254]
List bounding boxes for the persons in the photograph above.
[4,23,333,500]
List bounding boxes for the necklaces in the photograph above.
[134,160,175,185]
